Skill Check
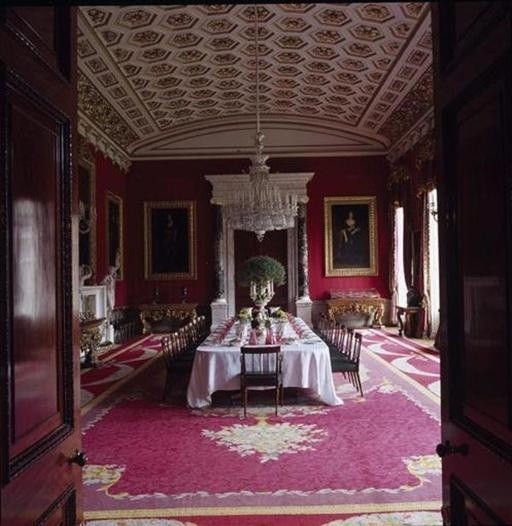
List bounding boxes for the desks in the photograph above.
[137,297,200,336]
[324,296,388,331]
[395,304,422,338]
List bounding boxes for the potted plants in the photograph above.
[230,254,289,325]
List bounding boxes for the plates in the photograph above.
[207,310,318,349]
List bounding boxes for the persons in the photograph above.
[342,211,361,243]
[160,212,178,239]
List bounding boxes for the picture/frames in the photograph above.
[141,199,200,283]
[105,187,125,284]
[76,134,97,287]
[321,193,380,279]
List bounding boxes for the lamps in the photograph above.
[211,3,303,247]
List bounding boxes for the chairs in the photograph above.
[154,306,365,419]
